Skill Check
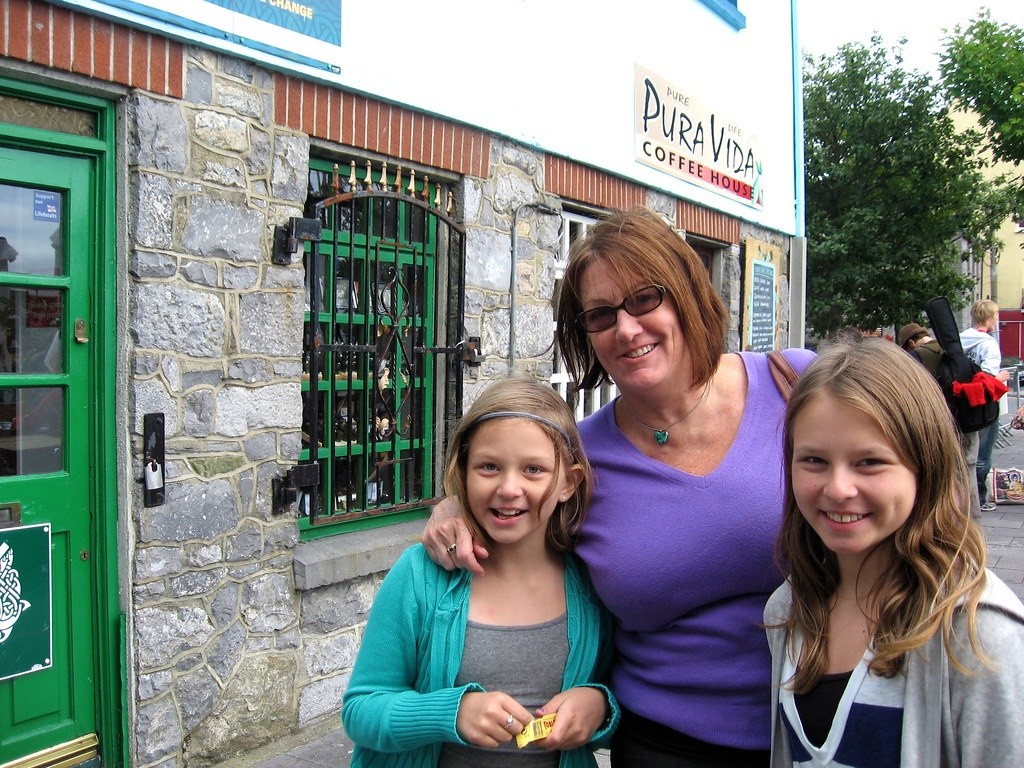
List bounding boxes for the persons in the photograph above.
[763,338,1024,768]
[899,323,981,518]
[421,209,820,768]
[341,380,621,768]
[959,299,1010,510]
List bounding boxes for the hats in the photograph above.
[897,323,929,346]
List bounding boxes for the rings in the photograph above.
[503,715,514,729]
[447,544,456,555]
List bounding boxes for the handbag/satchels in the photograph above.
[985,468,1024,505]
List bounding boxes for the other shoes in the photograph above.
[980,502,996,511]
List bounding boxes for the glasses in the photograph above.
[574,284,667,333]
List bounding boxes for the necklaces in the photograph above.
[630,381,709,445]
